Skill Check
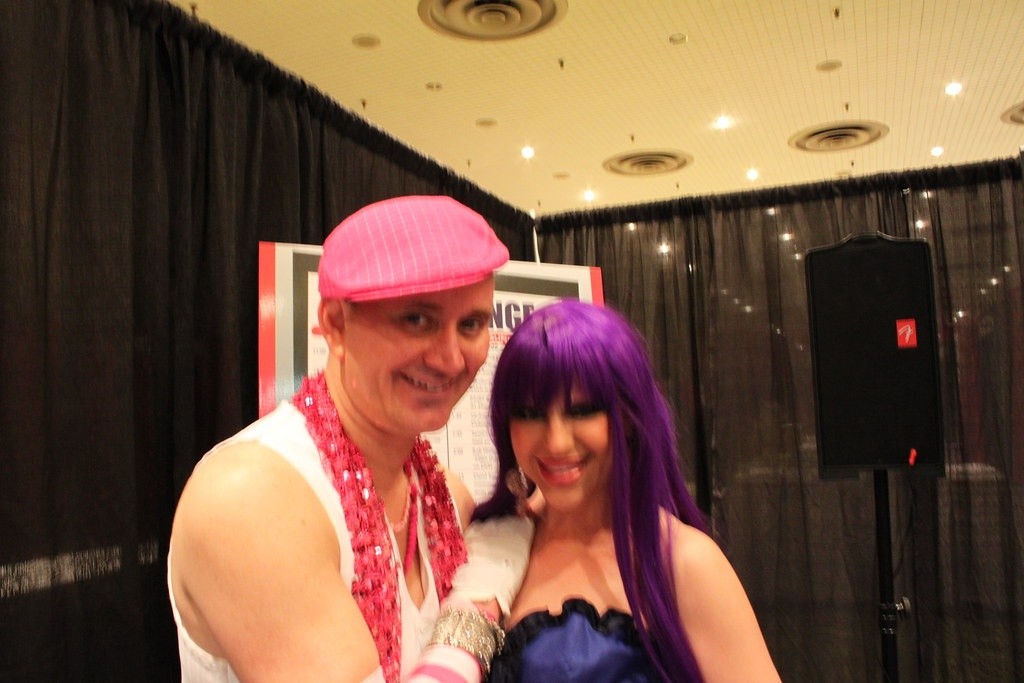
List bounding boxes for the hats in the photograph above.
[318,194,512,301]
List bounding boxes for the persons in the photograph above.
[471,301,783,683]
[167,198,536,683]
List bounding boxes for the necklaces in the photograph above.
[386,464,419,577]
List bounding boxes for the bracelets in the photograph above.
[429,611,501,673]
